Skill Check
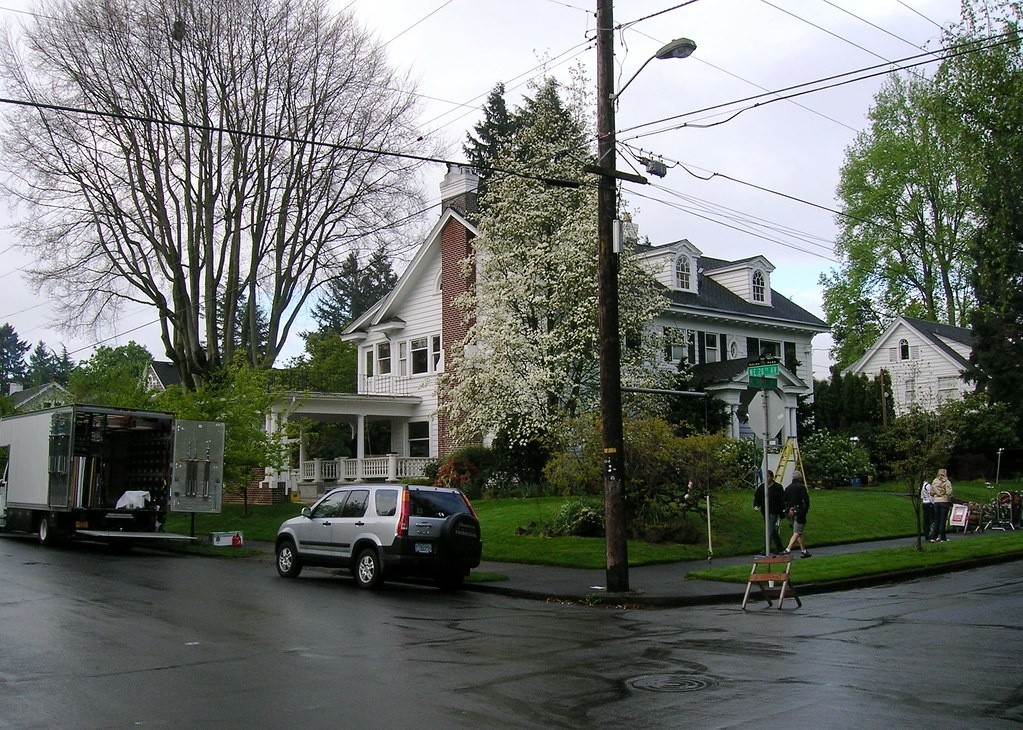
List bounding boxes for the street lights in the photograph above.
[591,37,699,593]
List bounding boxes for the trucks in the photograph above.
[0,402,225,553]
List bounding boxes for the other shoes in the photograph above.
[941,538,949,542]
[783,550,791,555]
[929,539,935,542]
[760,550,766,555]
[800,550,812,558]
[774,548,785,555]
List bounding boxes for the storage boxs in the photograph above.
[208,531,243,547]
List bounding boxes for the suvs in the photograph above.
[275,483,485,592]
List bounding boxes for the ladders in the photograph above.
[774,435,810,495]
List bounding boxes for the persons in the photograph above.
[753,470,813,558]
[921,469,954,543]
